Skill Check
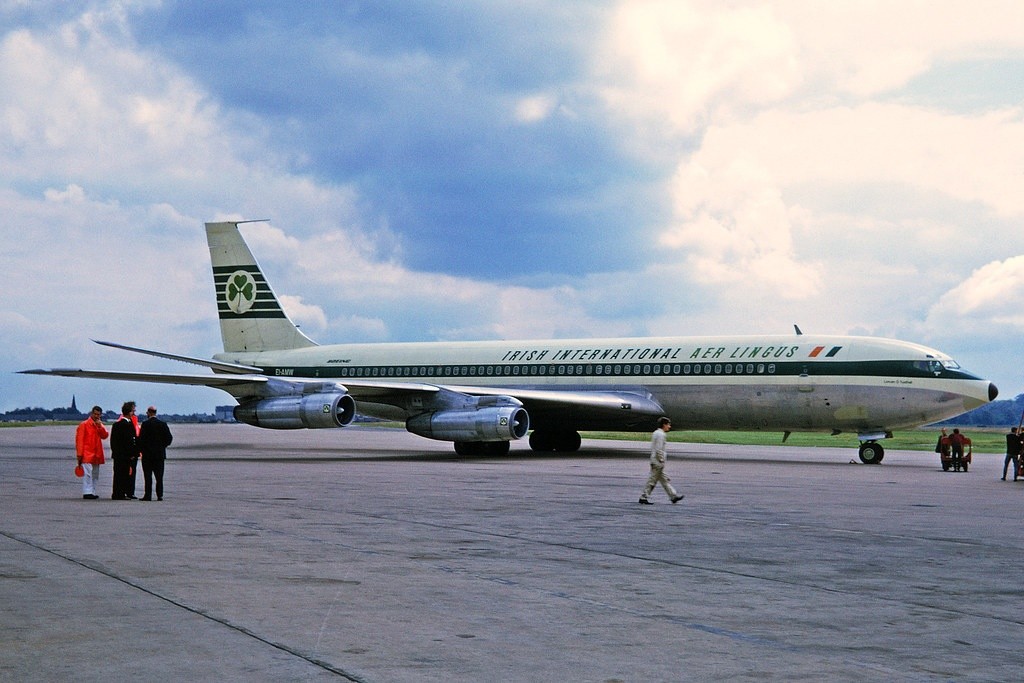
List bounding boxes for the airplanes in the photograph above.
[13,218,999,466]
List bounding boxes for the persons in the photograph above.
[75,406,109,499]
[935,428,964,472]
[110,401,172,501]
[1000,426,1024,482]
[638,417,685,504]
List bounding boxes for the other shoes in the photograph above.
[953,469,960,472]
[1014,479,1017,482]
[1001,478,1005,481]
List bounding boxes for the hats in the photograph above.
[941,428,947,431]
[75,465,85,477]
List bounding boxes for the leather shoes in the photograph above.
[112,495,131,500]
[639,499,654,505]
[83,494,99,499]
[127,495,138,499]
[157,497,163,501]
[139,496,151,501]
[671,495,684,503]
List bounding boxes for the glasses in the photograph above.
[146,412,150,414]
